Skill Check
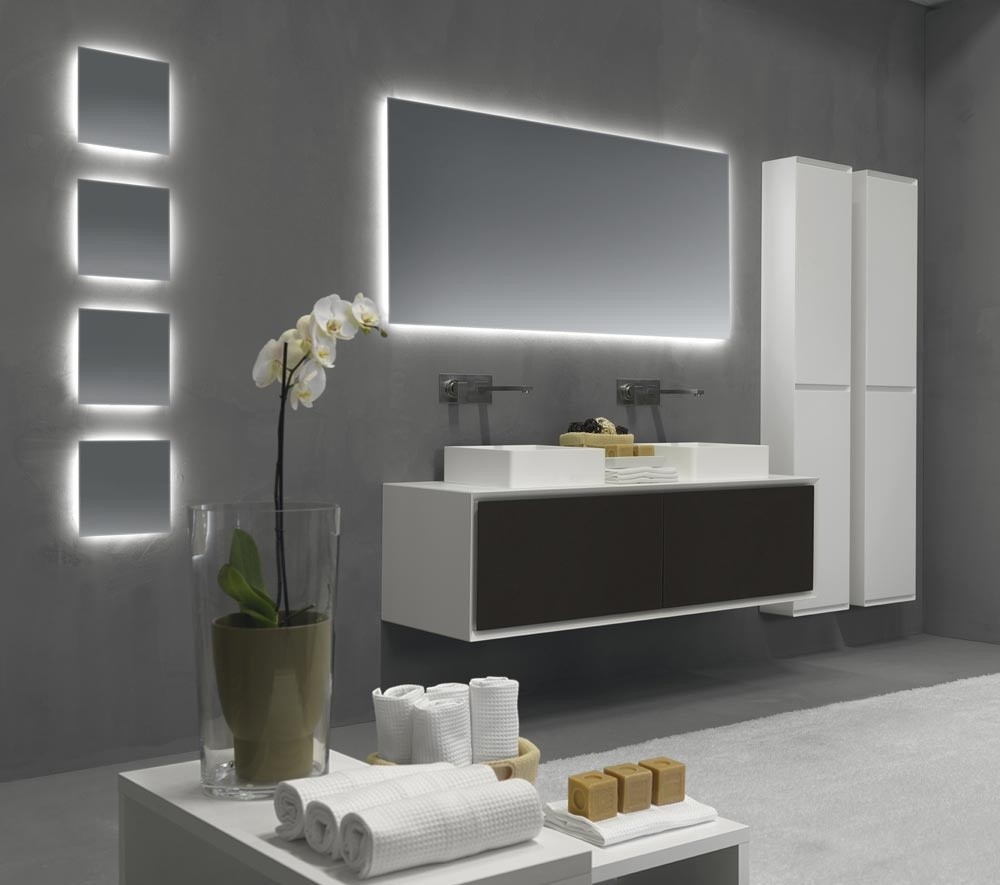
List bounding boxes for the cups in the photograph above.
[184,499,343,802]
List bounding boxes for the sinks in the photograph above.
[644,441,771,480]
[443,443,605,488]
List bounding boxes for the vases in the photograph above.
[185,494,339,793]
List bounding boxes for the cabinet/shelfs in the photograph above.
[380,465,817,651]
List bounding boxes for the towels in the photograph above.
[270,761,457,843]
[334,778,543,880]
[542,792,717,848]
[368,673,523,768]
[301,762,498,858]
[606,468,679,485]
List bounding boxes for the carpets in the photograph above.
[536,671,1000,885]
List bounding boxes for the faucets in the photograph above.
[645,387,705,396]
[476,380,534,395]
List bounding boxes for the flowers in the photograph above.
[220,293,390,625]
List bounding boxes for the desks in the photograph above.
[117,740,592,885]
[541,812,747,885]
